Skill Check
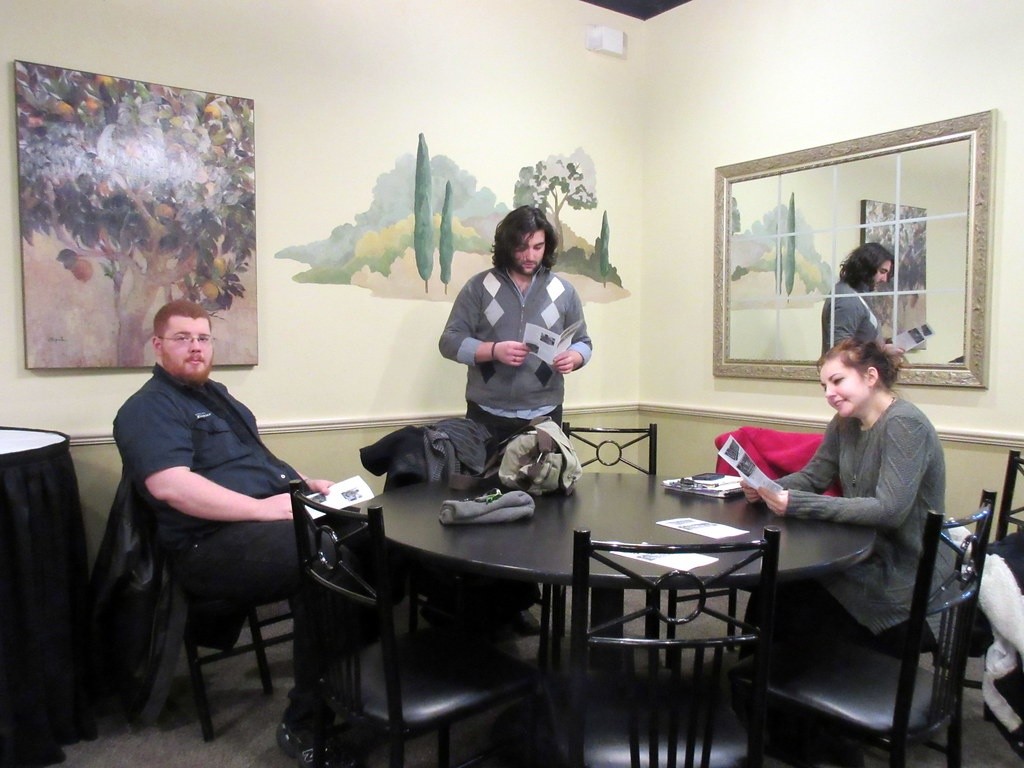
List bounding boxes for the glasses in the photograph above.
[159,335,216,344]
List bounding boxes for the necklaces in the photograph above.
[852,396,895,487]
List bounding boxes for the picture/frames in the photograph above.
[0,428,93,768]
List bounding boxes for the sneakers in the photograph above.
[515,611,541,634]
[276,720,357,768]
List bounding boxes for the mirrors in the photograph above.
[713,110,999,391]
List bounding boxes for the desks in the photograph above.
[343,475,889,766]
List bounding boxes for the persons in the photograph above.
[740,340,946,767]
[438,206,593,637]
[820,241,907,370]
[111,301,409,768]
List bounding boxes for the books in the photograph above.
[662,473,744,498]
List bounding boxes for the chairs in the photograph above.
[730,487,992,768]
[105,443,309,740]
[549,422,661,638]
[555,528,789,768]
[272,494,537,767]
[715,422,837,497]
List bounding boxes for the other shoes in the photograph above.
[764,735,866,768]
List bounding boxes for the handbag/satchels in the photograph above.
[483,415,582,496]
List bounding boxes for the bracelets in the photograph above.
[491,340,501,363]
